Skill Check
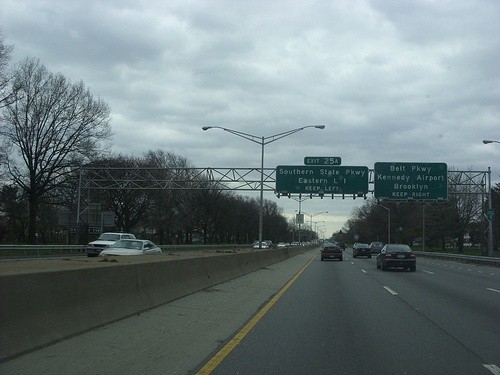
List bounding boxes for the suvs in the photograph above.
[86,232,137,258]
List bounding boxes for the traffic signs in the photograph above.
[275,165,368,195]
[374,161,448,201]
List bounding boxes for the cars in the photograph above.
[369,241,384,254]
[98,237,163,258]
[252,238,348,250]
[321,245,343,261]
[352,243,371,258]
[352,242,361,249]
[376,244,417,272]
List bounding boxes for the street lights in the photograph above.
[201,124,326,250]
[366,199,391,244]
[295,209,329,241]
